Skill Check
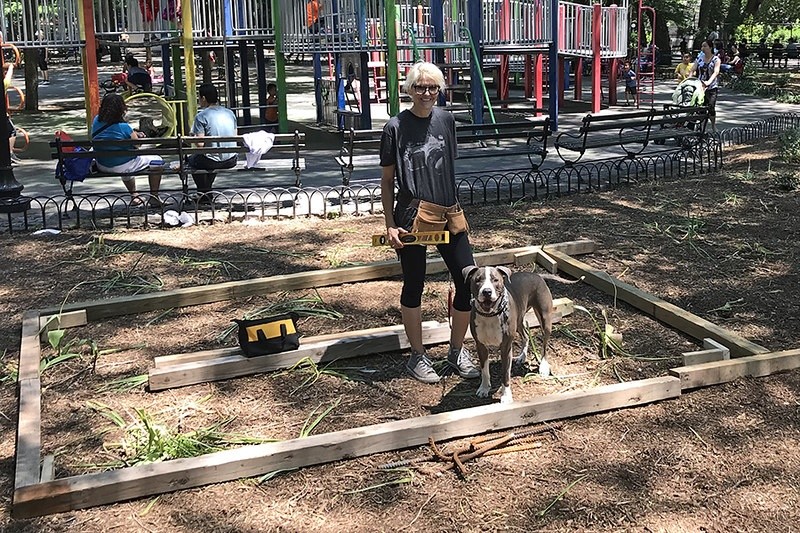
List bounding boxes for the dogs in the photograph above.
[462,265,587,406]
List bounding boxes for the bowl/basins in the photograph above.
[149,160,166,170]
[169,161,180,169]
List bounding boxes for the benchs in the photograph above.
[725,51,751,79]
[753,45,788,69]
[555,104,711,184]
[49,130,306,205]
[334,117,552,204]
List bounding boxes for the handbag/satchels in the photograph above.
[111,73,127,86]
[56,146,93,181]
[231,313,299,358]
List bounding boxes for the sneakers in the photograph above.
[406,350,440,383]
[447,343,481,378]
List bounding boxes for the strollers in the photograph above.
[654,77,705,147]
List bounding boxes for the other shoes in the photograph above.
[712,126,718,131]
[10,154,20,161]
[131,197,146,205]
[151,200,170,207]
[39,80,50,85]
[633,103,638,107]
[625,102,630,105]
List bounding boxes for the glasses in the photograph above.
[413,84,440,95]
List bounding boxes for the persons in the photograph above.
[122,53,154,95]
[138,0,182,42]
[35,30,50,85]
[636,42,663,81]
[689,39,721,133]
[7,113,21,164]
[264,83,278,134]
[757,38,769,68]
[678,38,688,54]
[739,38,751,66]
[197,32,218,84]
[379,59,480,383]
[187,83,237,203]
[675,52,695,81]
[619,62,638,107]
[784,38,799,68]
[721,38,743,75]
[306,0,325,34]
[91,93,173,207]
[772,39,785,69]
